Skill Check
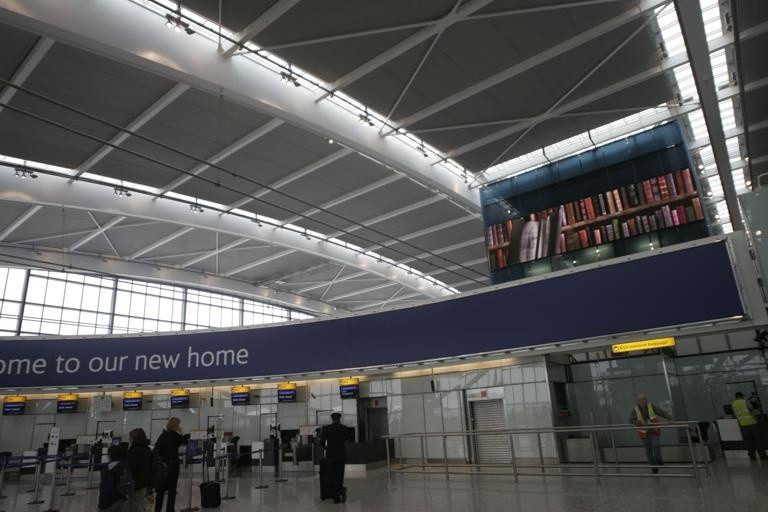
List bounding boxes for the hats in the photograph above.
[637,393,649,399]
[331,413,341,418]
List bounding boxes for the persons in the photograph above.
[320,412,349,504]
[731,392,768,460]
[628,393,673,474]
[125,428,155,512]
[154,416,190,511]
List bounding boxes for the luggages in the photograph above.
[319,460,333,500]
[199,480,221,508]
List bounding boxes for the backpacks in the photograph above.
[115,464,136,496]
[147,447,173,490]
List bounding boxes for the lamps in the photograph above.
[252,210,264,228]
[281,57,300,88]
[301,223,311,240]
[164,1,195,35]
[360,104,374,126]
[189,195,204,213]
[417,138,429,158]
[14,160,38,179]
[113,179,131,198]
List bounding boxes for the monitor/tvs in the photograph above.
[340,385,360,399]
[57,401,78,413]
[278,389,296,403]
[3,402,26,415]
[123,398,143,410]
[171,395,190,409]
[232,392,250,405]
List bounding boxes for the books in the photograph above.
[483,167,704,273]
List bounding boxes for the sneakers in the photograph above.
[334,486,347,503]
[652,468,658,474]
[750,453,768,461]
